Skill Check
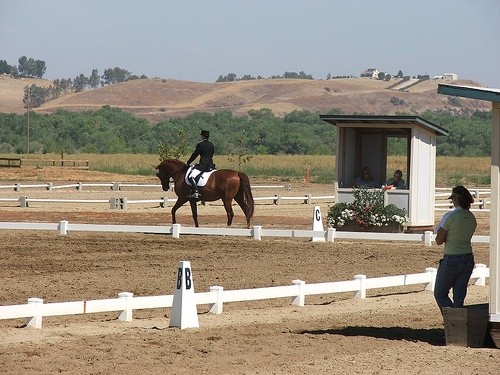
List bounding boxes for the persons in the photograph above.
[382,170,405,189]
[185,130,214,198]
[434,186,477,316]
[353,166,380,188]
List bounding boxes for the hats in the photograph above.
[199,130,210,137]
[448,185,474,203]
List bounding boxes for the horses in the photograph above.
[155,159,255,229]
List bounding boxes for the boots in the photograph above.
[187,176,199,199]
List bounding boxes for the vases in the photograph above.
[330,219,404,234]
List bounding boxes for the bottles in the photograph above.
[340,179,344,188]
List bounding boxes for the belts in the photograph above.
[442,253,473,258]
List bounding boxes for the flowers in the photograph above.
[326,185,410,228]
